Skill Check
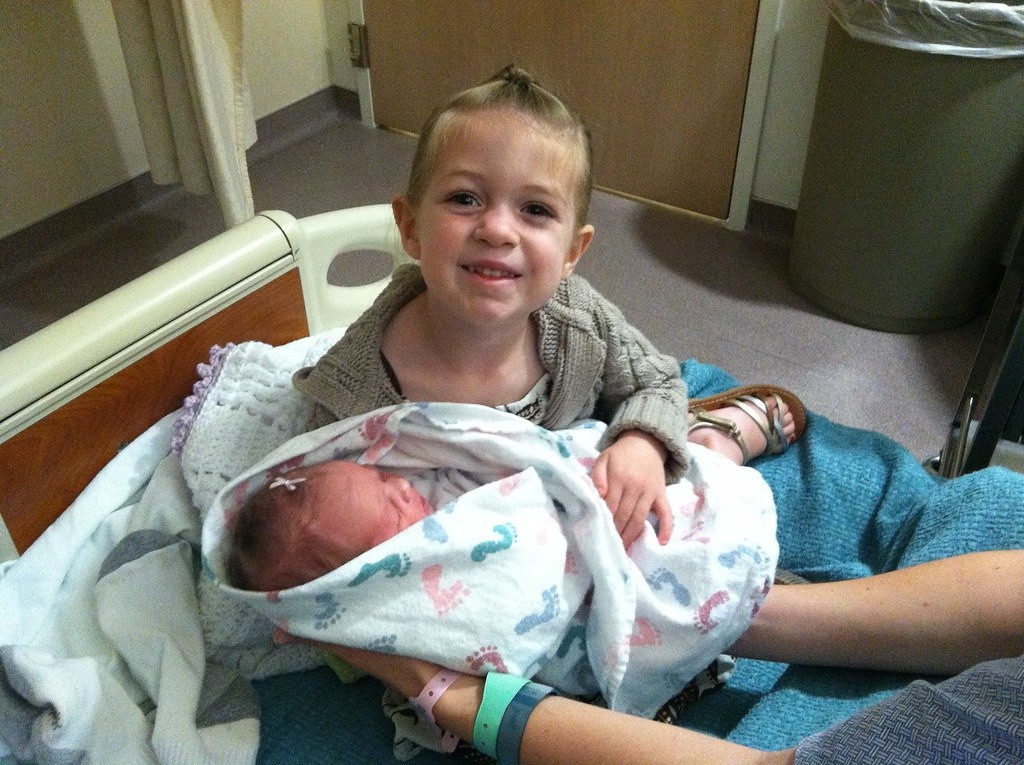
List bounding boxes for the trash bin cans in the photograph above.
[783,0,1024,335]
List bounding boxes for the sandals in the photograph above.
[688,384,807,467]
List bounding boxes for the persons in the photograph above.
[225,463,781,696]
[269,546,1024,765]
[305,64,799,555]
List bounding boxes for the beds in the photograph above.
[1,201,1024,764]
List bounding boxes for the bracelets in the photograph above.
[402,656,561,765]
